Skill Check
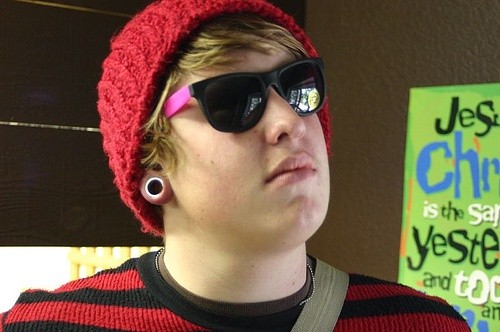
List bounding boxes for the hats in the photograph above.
[96,0,331,238]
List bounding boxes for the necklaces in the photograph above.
[156,248,315,306]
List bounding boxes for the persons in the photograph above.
[0,0,471,332]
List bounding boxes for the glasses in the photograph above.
[160,56,327,133]
